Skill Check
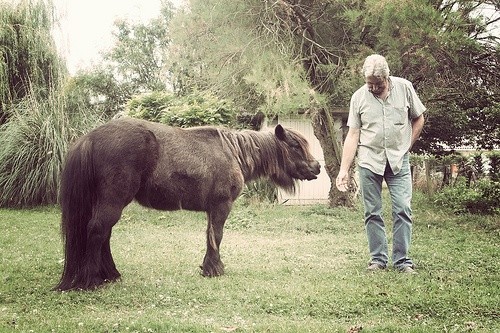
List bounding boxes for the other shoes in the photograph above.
[394,265,418,275]
[365,264,385,271]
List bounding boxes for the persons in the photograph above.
[335,53,427,273]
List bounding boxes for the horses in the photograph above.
[49,123,322,295]
